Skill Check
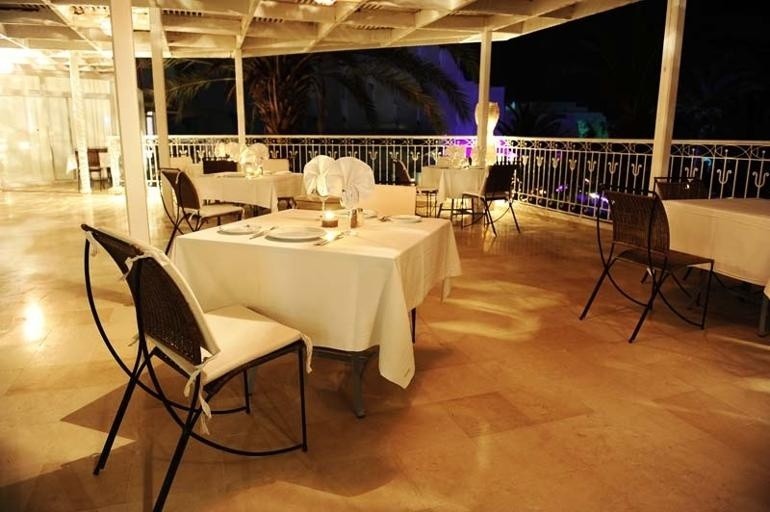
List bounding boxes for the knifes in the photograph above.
[249,225,280,241]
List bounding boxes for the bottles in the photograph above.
[350,207,364,228]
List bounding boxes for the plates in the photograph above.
[387,214,422,224]
[219,223,260,235]
[266,224,328,241]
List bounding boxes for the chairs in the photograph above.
[78,223,315,512]
[578,182,718,345]
[640,176,727,308]
